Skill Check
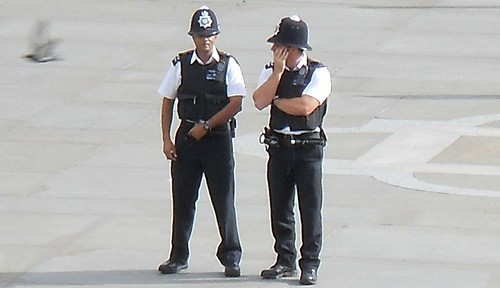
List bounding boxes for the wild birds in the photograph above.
[20,19,62,63]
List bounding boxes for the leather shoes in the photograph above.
[225,263,240,277]
[158,259,188,273]
[260,263,298,279]
[300,268,317,285]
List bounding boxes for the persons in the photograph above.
[158,7,247,277]
[253,15,331,284]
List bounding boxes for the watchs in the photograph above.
[200,120,213,136]
[271,94,280,105]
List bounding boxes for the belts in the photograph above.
[182,120,228,131]
[266,130,320,140]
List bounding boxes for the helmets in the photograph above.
[187,8,220,36]
[267,17,312,51]
